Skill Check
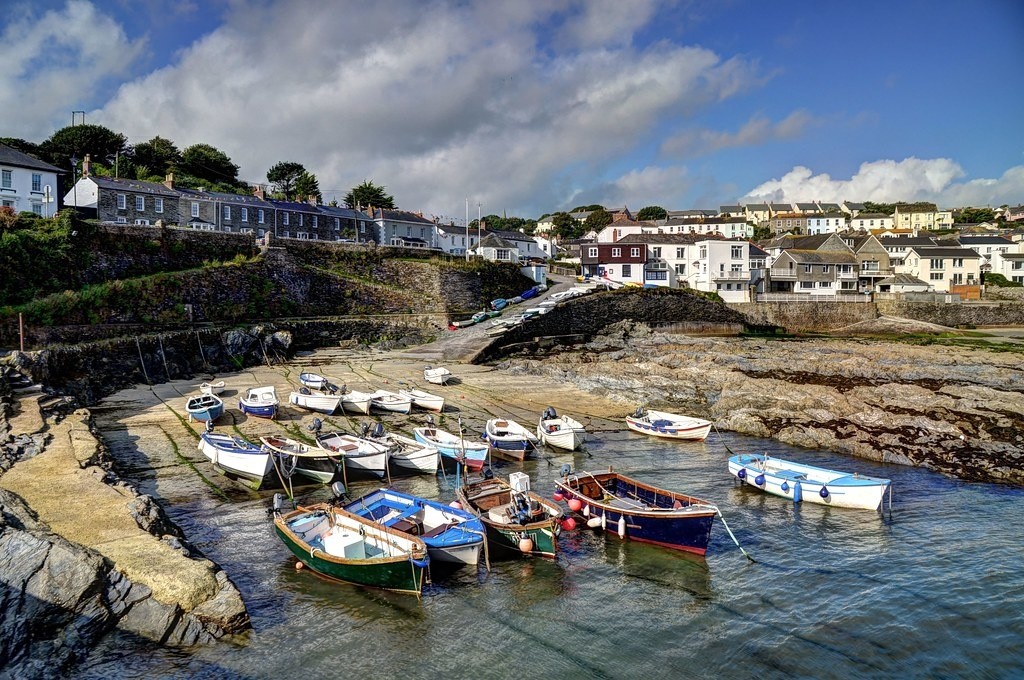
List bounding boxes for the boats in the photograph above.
[729,451,891,511]
[272,501,432,597]
[453,467,562,560]
[452,274,657,337]
[344,487,486,566]
[557,466,720,556]
[626,409,713,441]
[187,363,589,492]
